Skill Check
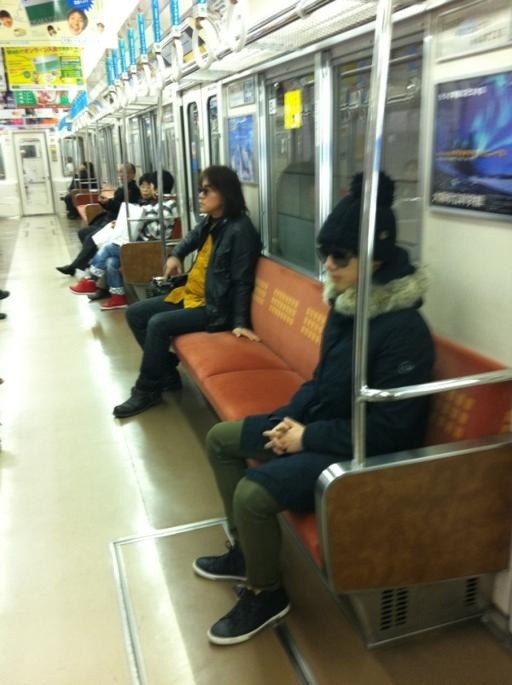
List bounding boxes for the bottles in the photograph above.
[317,171,396,262]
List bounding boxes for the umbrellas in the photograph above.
[88,290,108,300]
[112,387,162,418]
[56,266,75,276]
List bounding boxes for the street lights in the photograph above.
[111,202,147,247]
[91,220,116,249]
[146,273,188,299]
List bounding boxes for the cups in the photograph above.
[197,187,217,194]
[315,245,355,268]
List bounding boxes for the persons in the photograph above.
[111,163,265,418]
[97,24,104,32]
[0,10,12,28]
[47,26,56,37]
[0,288,10,321]
[194,211,434,647]
[57,152,178,312]
[68,9,88,35]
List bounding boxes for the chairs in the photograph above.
[68,279,96,295]
[99,294,128,310]
[132,378,183,394]
[192,540,248,582]
[206,585,291,646]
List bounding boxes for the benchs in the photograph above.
[74,185,512,650]
